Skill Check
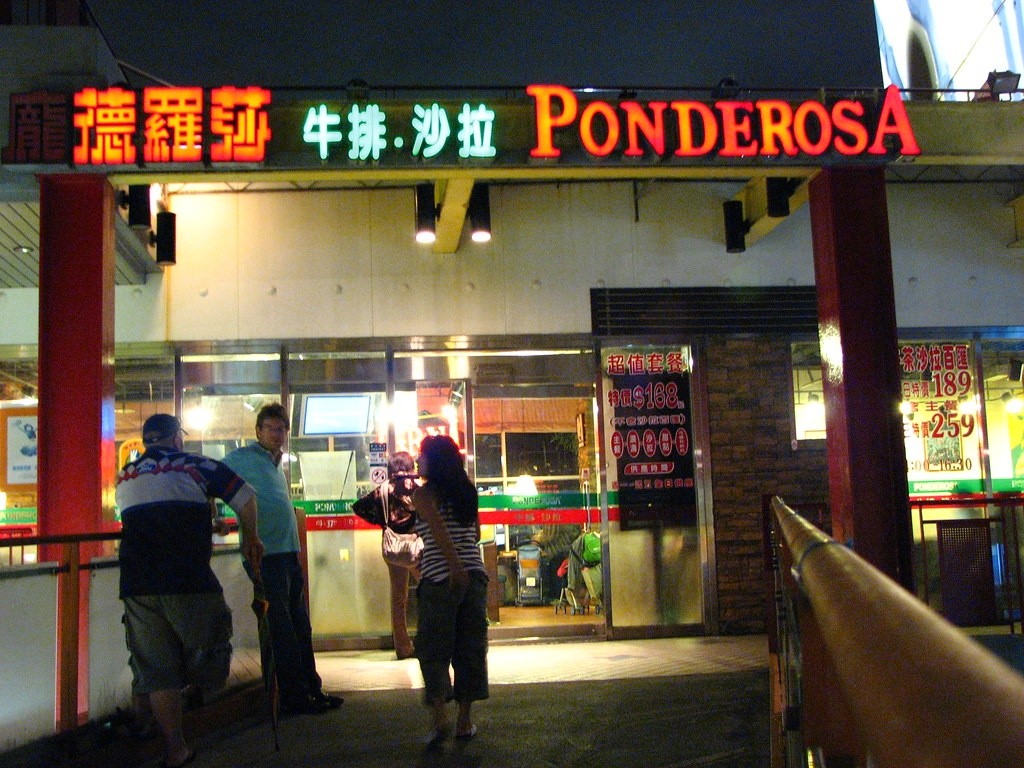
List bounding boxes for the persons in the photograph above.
[113,414,265,768]
[351,452,420,660]
[412,435,490,738]
[210,402,345,713]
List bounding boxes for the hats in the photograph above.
[142,414,189,444]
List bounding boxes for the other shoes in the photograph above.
[285,689,344,714]
[165,746,198,768]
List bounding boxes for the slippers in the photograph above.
[455,721,479,740]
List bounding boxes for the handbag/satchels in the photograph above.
[383,526,422,568]
